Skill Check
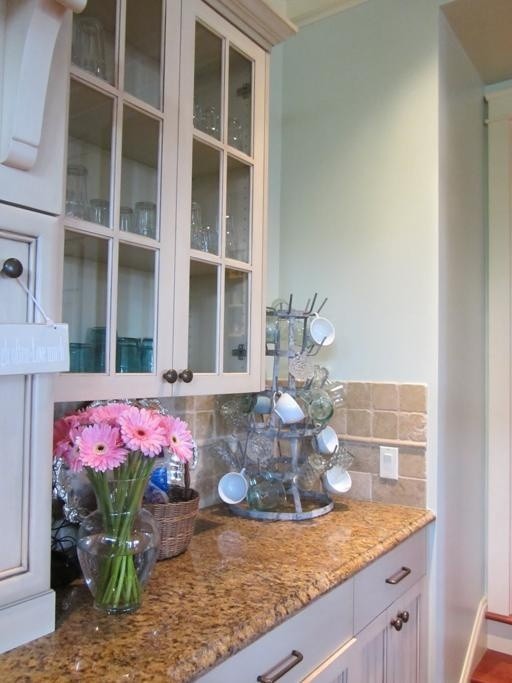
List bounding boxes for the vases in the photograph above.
[76,469,162,613]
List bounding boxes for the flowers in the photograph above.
[52,402,194,606]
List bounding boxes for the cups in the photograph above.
[67,165,156,237]
[71,15,106,78]
[206,299,356,512]
[69,326,153,373]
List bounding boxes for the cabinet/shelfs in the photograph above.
[192,526,429,683]
[0,199,64,656]
[54,0,299,401]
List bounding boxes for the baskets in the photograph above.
[136,461,199,562]
